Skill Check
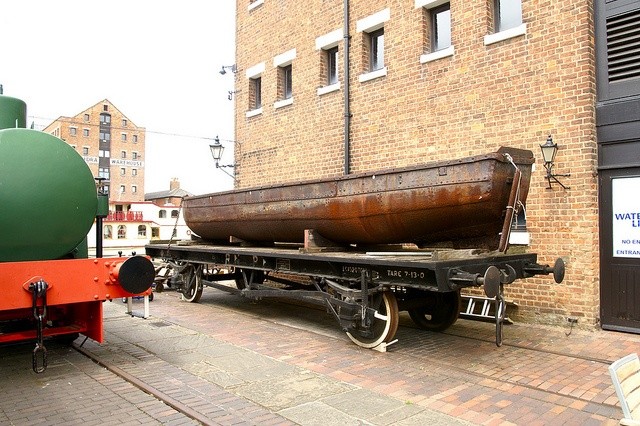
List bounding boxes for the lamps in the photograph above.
[538,133,571,191]
[209,135,237,182]
[228,90,238,101]
[219,65,237,75]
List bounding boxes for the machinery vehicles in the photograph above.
[144,146,566,355]
[0,85,156,374]
